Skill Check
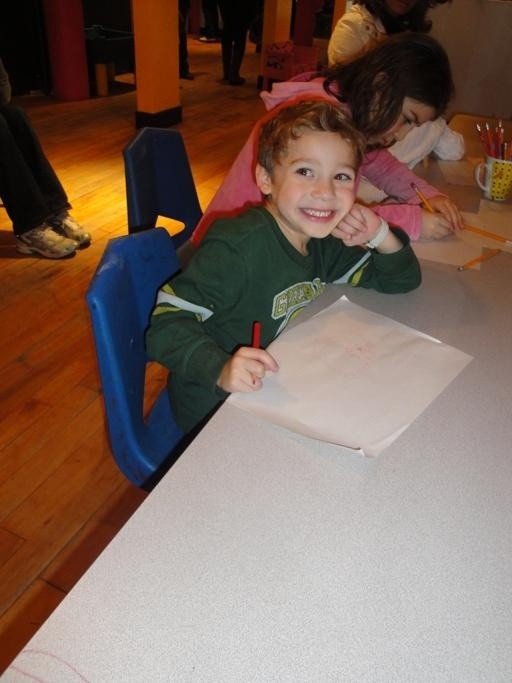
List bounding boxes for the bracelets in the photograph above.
[365,215,390,249]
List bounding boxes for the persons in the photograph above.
[143,99,422,438]
[181,0,264,85]
[175,30,466,273]
[327,0,453,68]
[1,65,91,260]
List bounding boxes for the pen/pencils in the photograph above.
[458,249,500,272]
[410,182,435,214]
[252,322,261,385]
[477,119,512,161]
[466,224,512,246]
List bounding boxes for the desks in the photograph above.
[0,113,512,681]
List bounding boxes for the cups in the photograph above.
[474,154,512,201]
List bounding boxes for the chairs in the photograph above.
[122,127,203,250]
[85,227,181,493]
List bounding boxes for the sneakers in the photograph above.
[230,76,244,85]
[15,223,78,258]
[199,36,215,41]
[180,71,193,80]
[48,212,90,247]
